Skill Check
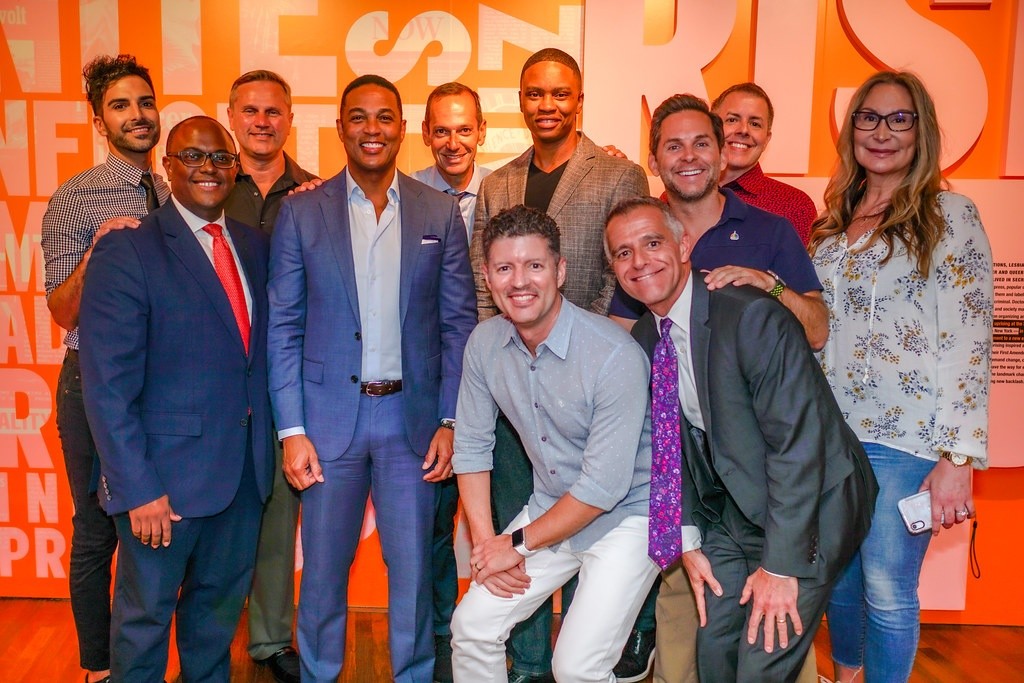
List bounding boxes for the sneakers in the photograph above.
[613,628,656,683]
[508,669,557,683]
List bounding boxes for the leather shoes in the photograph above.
[253,646,301,683]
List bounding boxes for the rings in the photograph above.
[776,619,787,623]
[475,563,481,571]
[956,508,969,517]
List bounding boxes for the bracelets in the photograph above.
[440,420,457,429]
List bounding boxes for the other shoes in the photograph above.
[85,672,113,683]
[433,631,453,683]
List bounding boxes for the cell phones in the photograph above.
[897,490,969,534]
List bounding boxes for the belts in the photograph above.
[361,379,402,397]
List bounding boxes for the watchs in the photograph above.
[765,270,787,296]
[511,527,537,558]
[938,451,973,468]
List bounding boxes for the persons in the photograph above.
[40,53,326,683]
[809,71,994,683]
[268,48,880,683]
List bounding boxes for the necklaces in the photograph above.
[860,190,887,222]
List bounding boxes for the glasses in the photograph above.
[168,151,237,169]
[851,111,917,132]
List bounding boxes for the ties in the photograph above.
[447,190,466,203]
[648,318,683,573]
[201,224,252,416]
